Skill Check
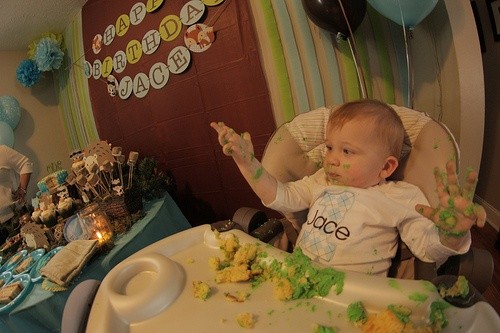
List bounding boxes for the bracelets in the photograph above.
[18,187,25,193]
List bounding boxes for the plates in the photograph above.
[64,215,84,243]
[0,247,67,315]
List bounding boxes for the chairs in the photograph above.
[232,99,482,308]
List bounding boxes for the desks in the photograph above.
[0,190,193,333]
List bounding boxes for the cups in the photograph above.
[81,203,114,246]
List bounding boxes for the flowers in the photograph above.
[15,29,65,89]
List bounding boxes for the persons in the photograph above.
[0,145,33,246]
[210,100,486,278]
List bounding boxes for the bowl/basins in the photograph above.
[0,234,23,253]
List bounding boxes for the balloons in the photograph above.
[0,96,21,148]
[367,0,439,27]
[300,0,366,38]
